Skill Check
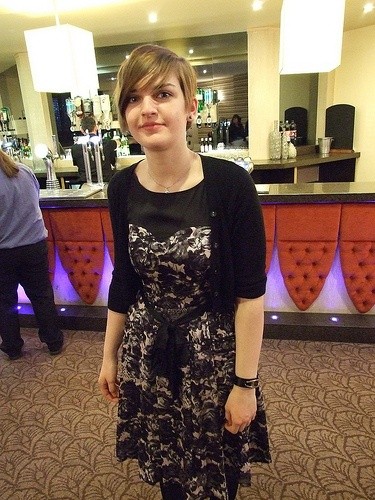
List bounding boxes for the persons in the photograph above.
[95,44,272,500]
[228,114,246,148]
[0,149,62,360]
[71,116,116,182]
[18,139,30,155]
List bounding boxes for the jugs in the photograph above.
[318,137,333,154]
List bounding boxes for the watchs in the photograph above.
[233,375,260,389]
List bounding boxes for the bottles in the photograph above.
[112,131,130,157]
[196,87,212,129]
[200,137,213,153]
[66,95,111,129]
[279,120,297,146]
[0,108,8,132]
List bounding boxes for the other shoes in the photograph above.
[1,350,20,359]
[47,331,63,354]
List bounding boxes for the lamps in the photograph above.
[33,142,60,189]
[76,136,93,184]
[280,0,346,76]
[90,135,103,185]
[22,13,100,97]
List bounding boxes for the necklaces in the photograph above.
[149,153,195,194]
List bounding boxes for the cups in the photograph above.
[297,137,302,146]
